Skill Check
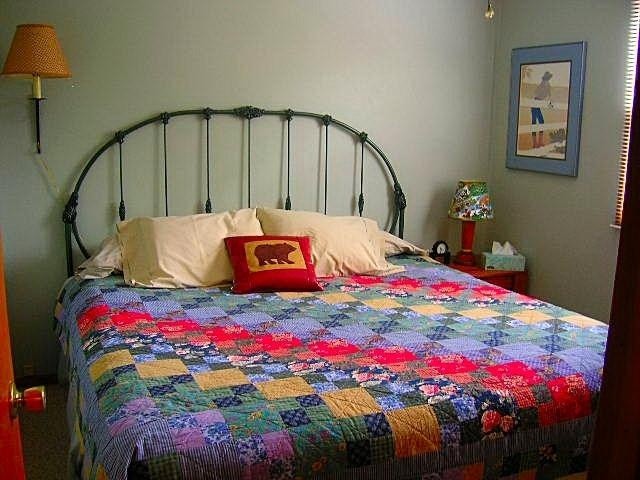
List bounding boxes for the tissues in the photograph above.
[480,241,526,272]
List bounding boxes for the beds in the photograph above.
[54,106,610,480]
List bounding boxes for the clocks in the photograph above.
[431,241,451,263]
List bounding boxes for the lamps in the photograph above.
[448,181,494,266]
[0,24,72,153]
[482,1,495,18]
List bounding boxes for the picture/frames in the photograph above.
[504,42,587,177]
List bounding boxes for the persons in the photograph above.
[529,69,556,149]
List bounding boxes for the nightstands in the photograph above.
[445,255,528,295]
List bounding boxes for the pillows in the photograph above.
[380,229,430,257]
[115,209,265,290]
[255,208,405,277]
[76,235,122,272]
[224,235,322,293]
